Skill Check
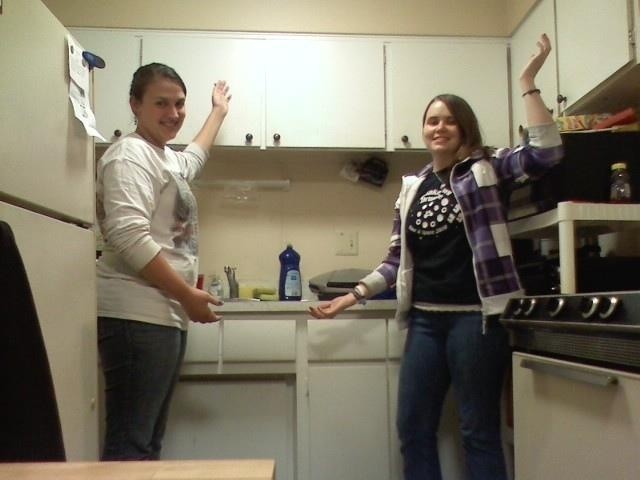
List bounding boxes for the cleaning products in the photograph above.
[207,273,223,297]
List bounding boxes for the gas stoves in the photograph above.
[499,283,640,338]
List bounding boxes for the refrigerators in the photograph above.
[1,0,103,462]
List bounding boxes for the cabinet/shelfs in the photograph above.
[179,314,298,375]
[297,311,475,479]
[65,25,138,150]
[385,35,513,154]
[510,1,640,149]
[140,30,387,153]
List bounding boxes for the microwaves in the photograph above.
[509,131,640,221]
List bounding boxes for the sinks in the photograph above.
[212,297,261,303]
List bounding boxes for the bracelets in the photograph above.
[348,288,367,305]
[520,88,541,97]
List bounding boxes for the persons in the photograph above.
[307,33,565,480]
[96,61,232,459]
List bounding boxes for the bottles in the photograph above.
[606,163,634,201]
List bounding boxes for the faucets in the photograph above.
[223,265,240,298]
